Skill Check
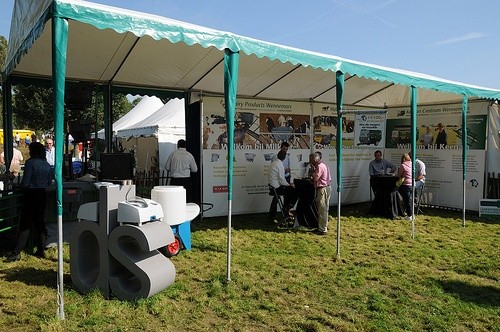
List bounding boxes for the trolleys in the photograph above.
[158,202,214,257]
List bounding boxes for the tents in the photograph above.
[93,94,186,182]
[0,0,499,320]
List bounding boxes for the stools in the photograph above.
[414,177,426,217]
[268,183,296,233]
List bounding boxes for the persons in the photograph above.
[267,150,300,218]
[309,151,331,235]
[165,140,199,203]
[420,121,448,149]
[316,115,354,136]
[397,153,415,218]
[1,131,55,178]
[368,150,405,217]
[398,150,426,207]
[267,141,292,223]
[3,141,54,262]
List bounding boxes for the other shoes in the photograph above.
[406,215,416,220]
[313,228,328,235]
[30,249,47,259]
[282,208,294,216]
[4,253,22,262]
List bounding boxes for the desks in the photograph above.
[293,178,321,229]
[370,173,406,222]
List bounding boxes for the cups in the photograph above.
[380,169,384,177]
